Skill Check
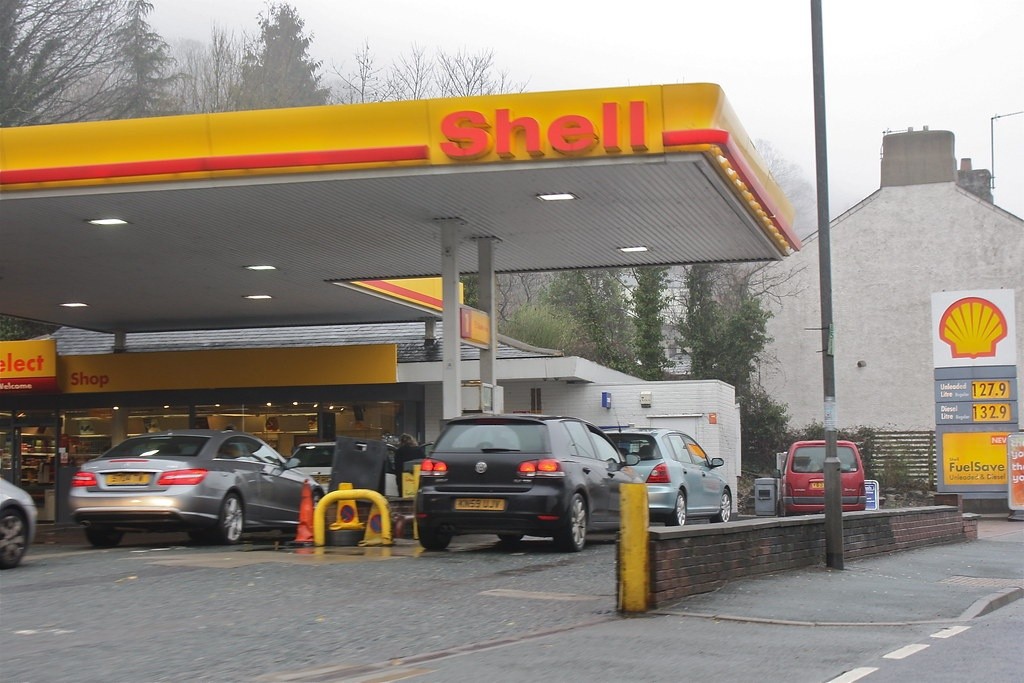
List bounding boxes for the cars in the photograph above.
[771,440,866,517]
[602,428,732,527]
[0,477,38,570]
[68,429,325,546]
[414,413,649,551]
[288,442,400,498]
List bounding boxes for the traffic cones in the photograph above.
[289,479,315,546]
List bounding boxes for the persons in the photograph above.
[395,433,425,486]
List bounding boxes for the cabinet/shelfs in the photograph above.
[20,433,57,499]
[68,412,318,465]
